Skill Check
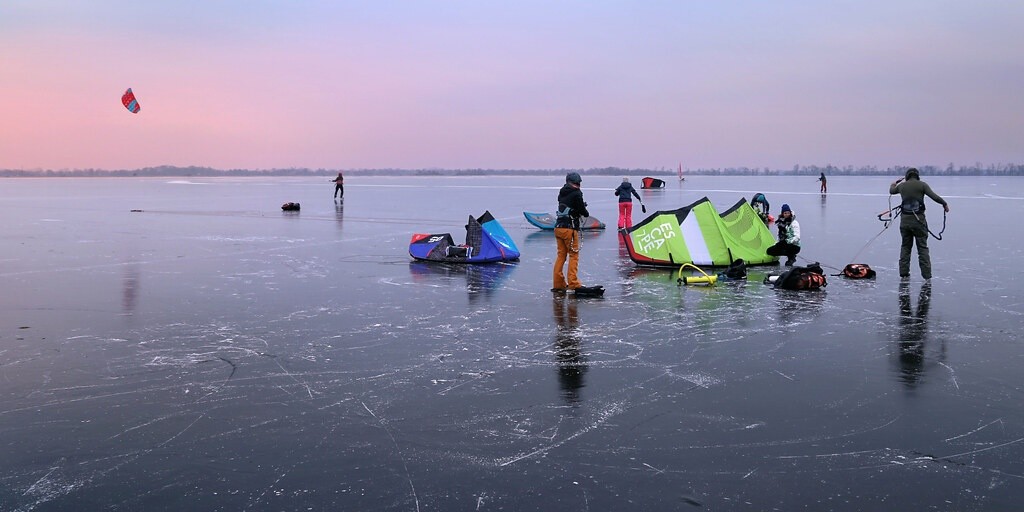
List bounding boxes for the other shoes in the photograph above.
[785,259,796,266]
[580,285,586,288]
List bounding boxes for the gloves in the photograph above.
[781,240,787,246]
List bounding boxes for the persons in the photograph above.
[897,284,940,399]
[615,178,642,231]
[751,193,770,217]
[553,173,589,292]
[766,203,801,266]
[328,173,344,200]
[818,173,827,193]
[618,231,635,279]
[334,200,344,223]
[553,295,589,405]
[889,168,949,280]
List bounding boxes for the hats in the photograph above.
[567,172,582,184]
[782,204,791,213]
